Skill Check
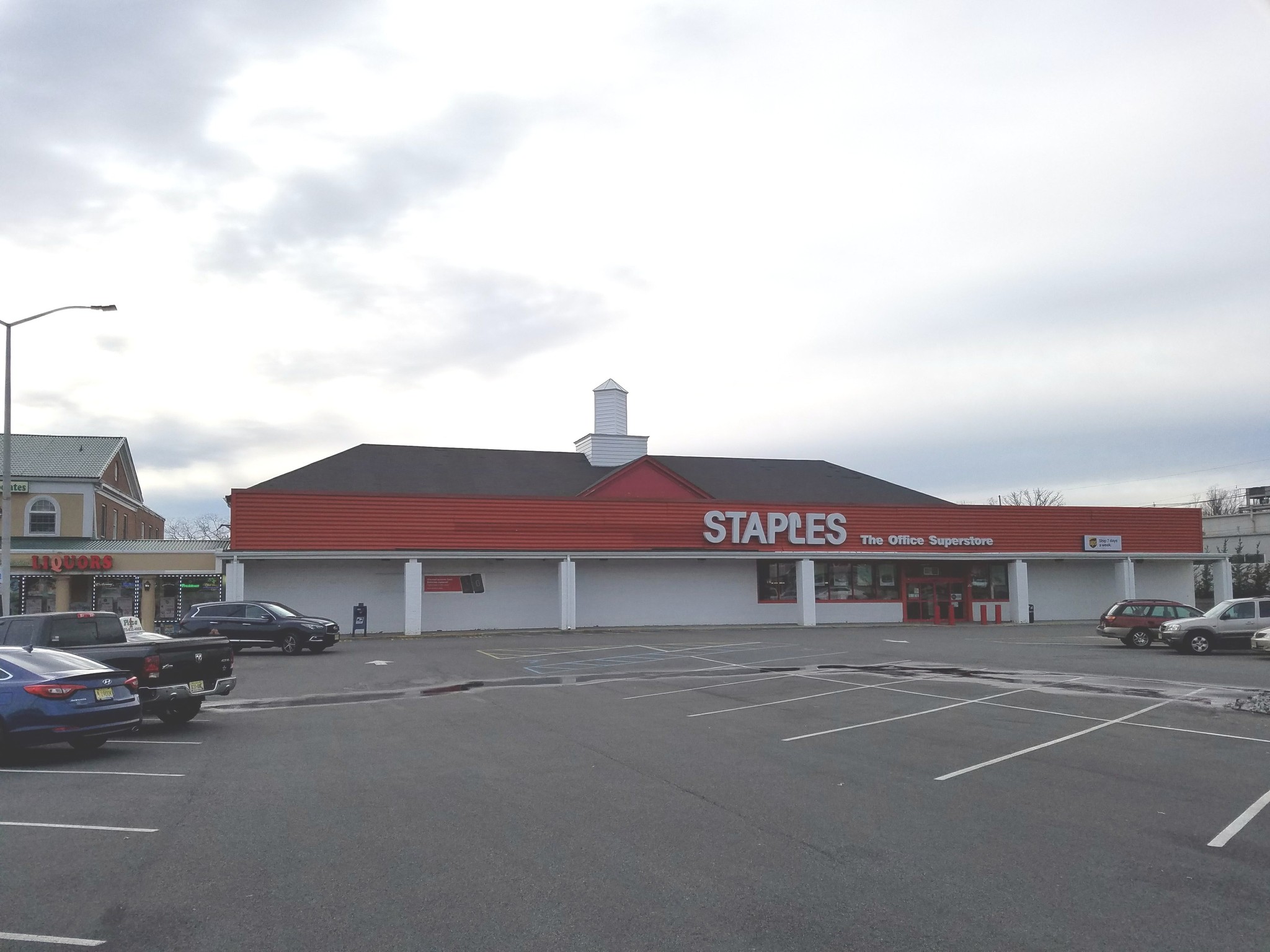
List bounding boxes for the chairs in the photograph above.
[1134,609,1147,616]
[1163,611,1172,619]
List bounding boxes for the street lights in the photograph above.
[0,305,117,617]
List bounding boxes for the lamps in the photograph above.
[144,581,151,591]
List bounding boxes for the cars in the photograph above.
[769,588,797,600]
[972,579,988,588]
[1251,626,1270,652]
[815,588,868,600]
[0,644,142,764]
[1096,599,1205,649]
[120,616,174,642]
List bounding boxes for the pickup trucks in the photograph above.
[0,611,237,725]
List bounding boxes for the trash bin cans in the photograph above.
[352,605,367,629]
[1028,604,1034,624]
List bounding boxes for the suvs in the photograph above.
[1158,595,1270,655]
[179,599,341,655]
